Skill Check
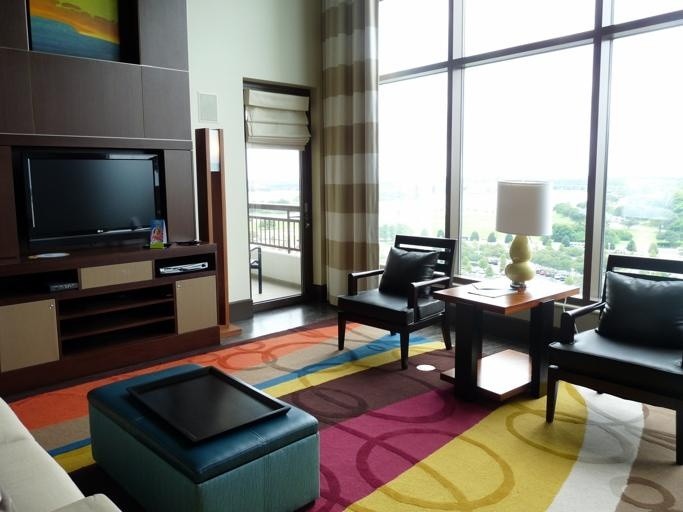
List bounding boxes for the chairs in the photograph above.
[546,254,683,465]
[338,235,459,370]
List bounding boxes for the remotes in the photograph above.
[143,242,171,248]
[175,240,198,244]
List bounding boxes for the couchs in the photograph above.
[0,397,122,512]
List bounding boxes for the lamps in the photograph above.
[495,180,553,288]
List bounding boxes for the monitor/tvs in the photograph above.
[23,150,162,250]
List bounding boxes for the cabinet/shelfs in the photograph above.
[0,241,221,396]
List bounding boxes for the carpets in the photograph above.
[7,314,683,512]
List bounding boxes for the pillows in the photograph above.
[595,271,683,349]
[379,245,441,298]
